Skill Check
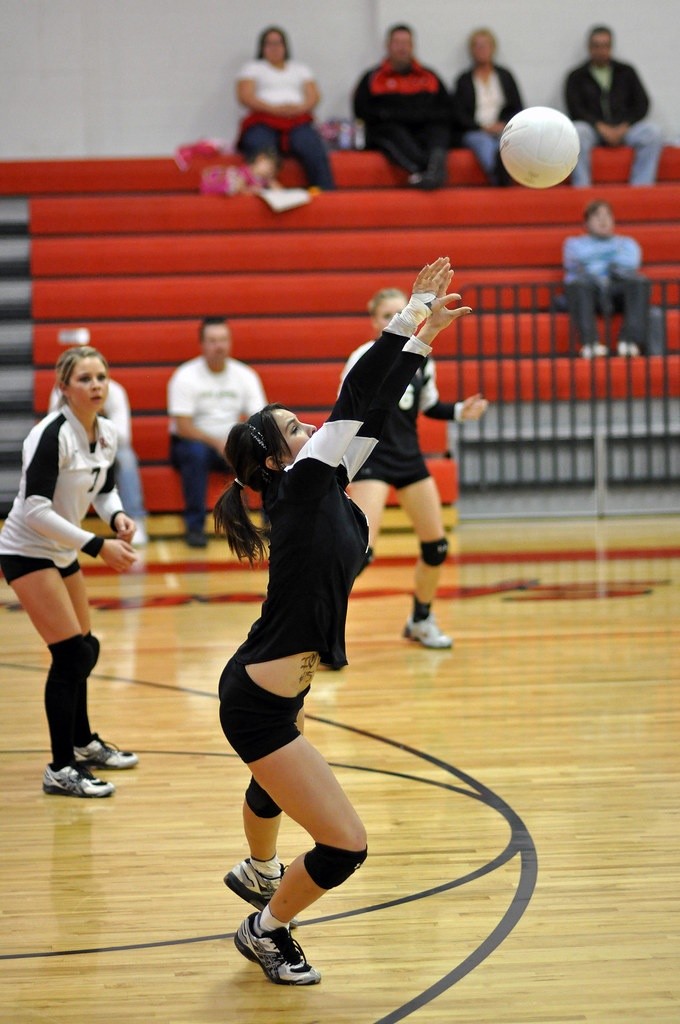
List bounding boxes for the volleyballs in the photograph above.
[500,106,581,189]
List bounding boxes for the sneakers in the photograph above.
[233,912,321,985]
[404,617,451,648]
[73,732,138,770]
[43,763,114,798]
[223,858,298,928]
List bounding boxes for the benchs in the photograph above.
[0,144,680,512]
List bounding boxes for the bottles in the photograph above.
[353,118,364,149]
[338,120,352,150]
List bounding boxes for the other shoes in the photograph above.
[583,341,638,358]
[186,530,207,547]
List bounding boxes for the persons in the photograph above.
[0,349,139,798]
[49,348,149,548]
[454,31,526,188]
[564,198,649,360]
[169,318,270,549]
[352,24,453,188]
[240,27,335,190]
[209,256,488,986]
[564,26,662,187]
[340,290,490,649]
